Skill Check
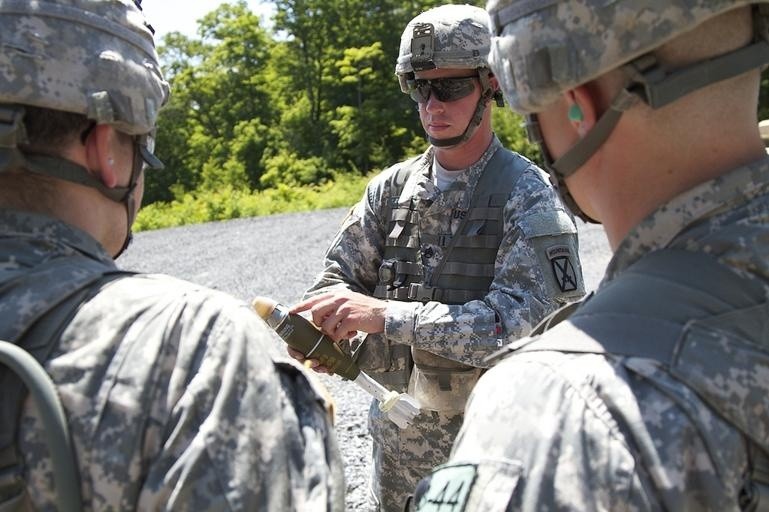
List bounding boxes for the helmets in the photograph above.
[492,0,767,114]
[396,6,491,72]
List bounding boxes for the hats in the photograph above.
[0,0,169,135]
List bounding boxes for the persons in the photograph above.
[289,0,584,512]
[1,0,345,511]
[415,0,769,511]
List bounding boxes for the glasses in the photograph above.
[396,77,485,102]
